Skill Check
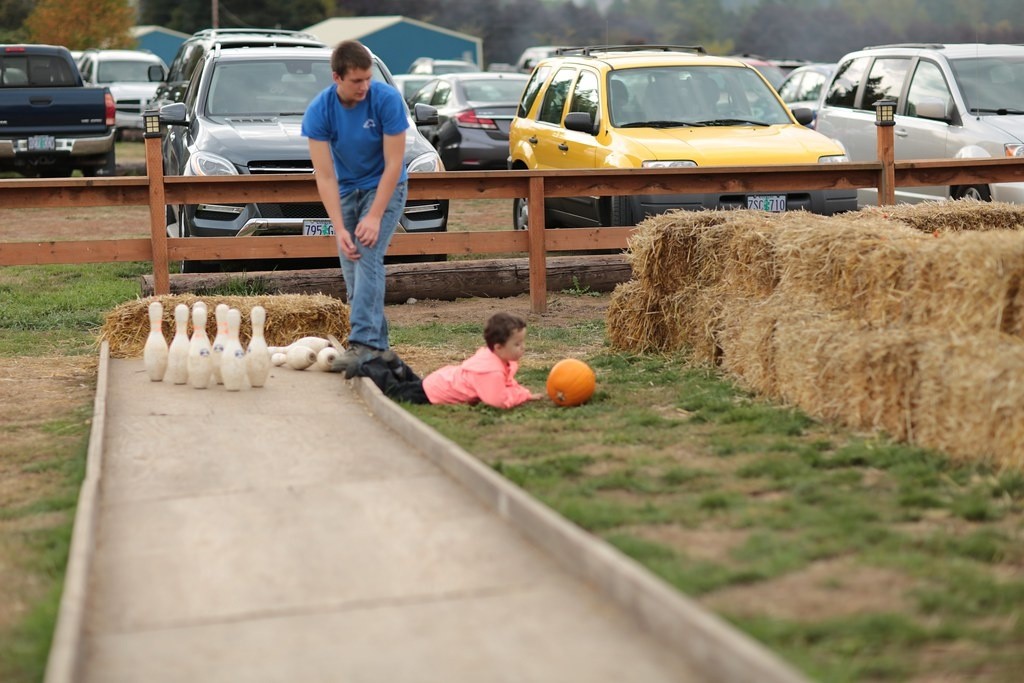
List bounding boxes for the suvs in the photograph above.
[502,44,858,235]
[159,41,451,268]
[812,38,1024,216]
[143,28,326,145]
[77,46,173,138]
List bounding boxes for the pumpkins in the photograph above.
[546,359,595,404]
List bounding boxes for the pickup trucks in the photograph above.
[0,42,121,174]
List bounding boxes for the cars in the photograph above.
[411,71,532,168]
[736,52,806,90]
[512,47,568,70]
[776,61,838,123]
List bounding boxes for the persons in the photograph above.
[345,312,543,409]
[302,40,411,370]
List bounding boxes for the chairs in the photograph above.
[610,79,645,122]
[1,68,29,86]
[683,77,720,115]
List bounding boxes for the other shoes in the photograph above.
[332,342,383,370]
[345,351,401,380]
